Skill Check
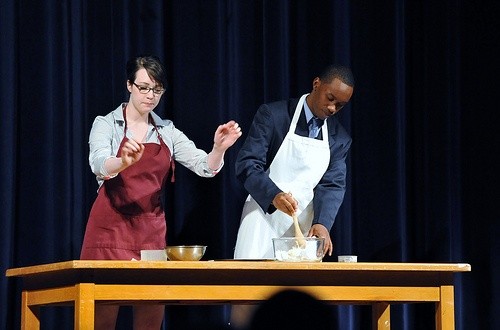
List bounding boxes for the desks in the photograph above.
[5,259,471,330]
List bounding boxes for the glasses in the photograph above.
[133,82,166,94]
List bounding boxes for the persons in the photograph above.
[80,54,242,330]
[230,63,353,330]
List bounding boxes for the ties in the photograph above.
[308,118,319,139]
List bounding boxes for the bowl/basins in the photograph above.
[272,237,325,262]
[164,245,208,261]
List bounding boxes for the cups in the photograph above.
[338,255,358,263]
